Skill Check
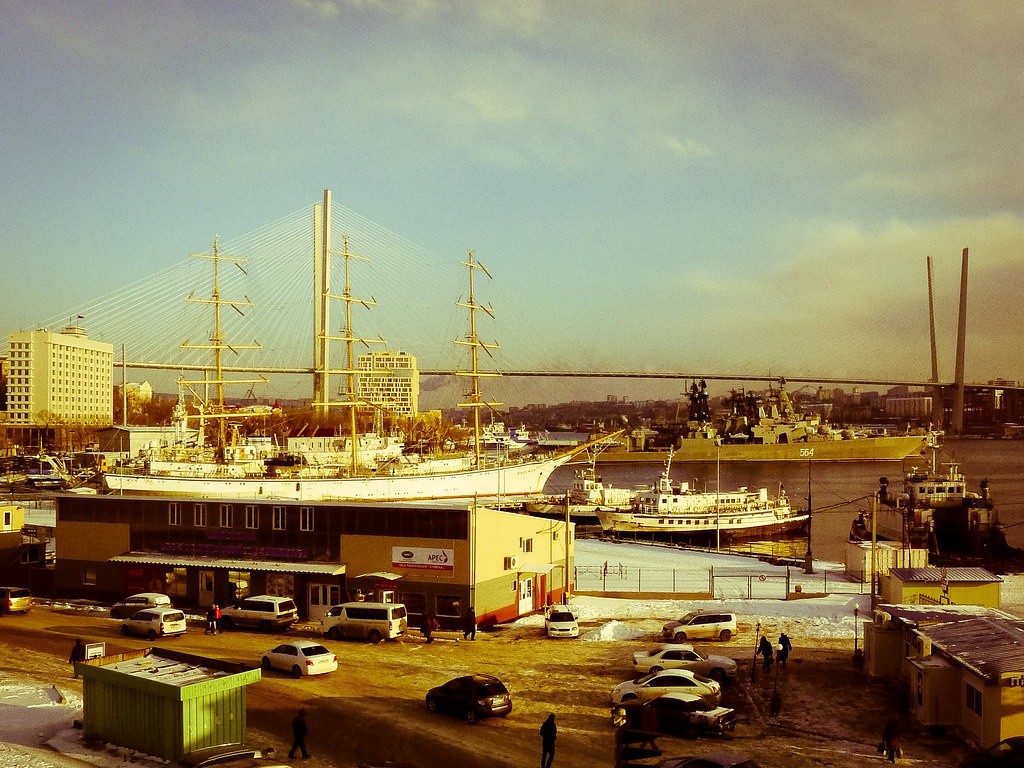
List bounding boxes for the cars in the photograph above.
[611,693,737,740]
[609,668,722,705]
[633,644,738,682]
[426,674,513,724]
[544,612,579,638]
[262,641,338,679]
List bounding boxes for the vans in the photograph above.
[321,602,408,644]
[663,611,737,644]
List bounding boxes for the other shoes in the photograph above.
[425,638,433,643]
[471,638,475,641]
[463,634,467,640]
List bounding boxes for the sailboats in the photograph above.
[103,190,627,510]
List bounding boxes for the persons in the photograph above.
[421,608,433,643]
[540,714,556,768]
[463,607,476,640]
[882,719,902,763]
[288,708,311,759]
[757,636,772,671]
[69,638,86,678]
[205,604,223,635]
[779,633,792,666]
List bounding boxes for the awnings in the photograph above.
[105,552,345,575]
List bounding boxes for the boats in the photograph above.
[553,376,927,462]
[545,424,574,432]
[596,443,809,544]
[851,431,1024,568]
[524,442,654,525]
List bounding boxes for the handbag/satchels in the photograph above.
[877,743,885,754]
[433,620,439,630]
[896,746,904,759]
[475,624,478,630]
[769,654,774,664]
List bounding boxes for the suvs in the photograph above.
[220,595,299,632]
[0,587,33,613]
[109,593,173,618]
[121,608,188,641]
